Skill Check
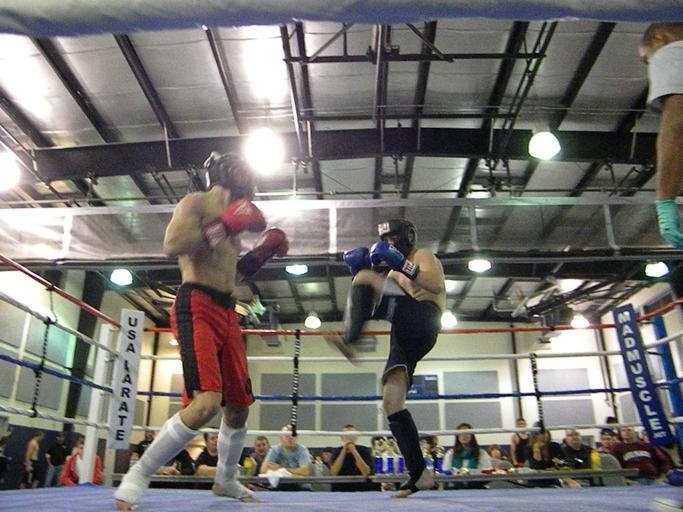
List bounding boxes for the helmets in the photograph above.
[376,219,416,257]
[206,150,257,197]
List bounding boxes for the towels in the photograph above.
[257,467,292,489]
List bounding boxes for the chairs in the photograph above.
[590,453,633,486]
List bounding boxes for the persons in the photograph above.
[637,21,683,486]
[323,217,447,497]
[113,153,289,511]
[0,422,104,487]
[126,418,681,487]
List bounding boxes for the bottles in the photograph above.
[128,452,140,470]
[314,457,323,477]
[590,448,602,469]
[244,455,253,476]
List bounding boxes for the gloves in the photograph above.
[369,239,418,280]
[654,198,683,249]
[237,228,288,277]
[340,247,369,276]
[202,197,267,249]
